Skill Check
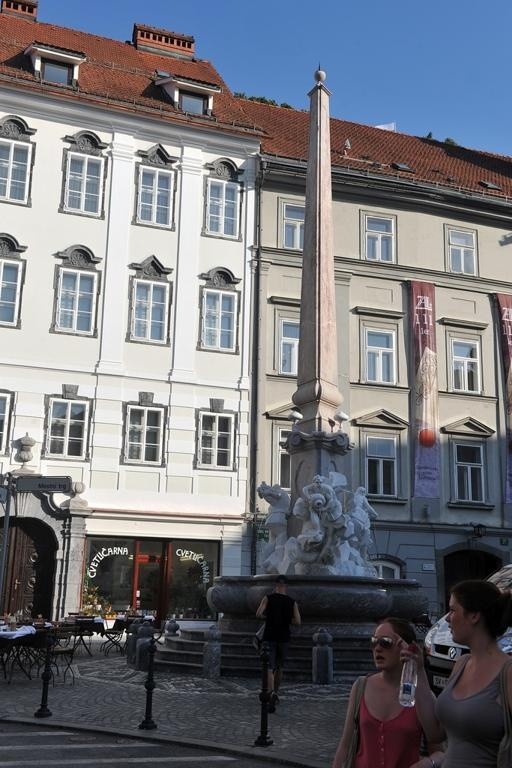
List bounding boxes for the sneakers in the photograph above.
[267,688,281,715]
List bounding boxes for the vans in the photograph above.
[422,564,512,704]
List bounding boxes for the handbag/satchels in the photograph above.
[340,740,359,768]
[497,734,511,767]
[254,624,266,649]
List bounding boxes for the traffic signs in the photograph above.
[14,472,71,493]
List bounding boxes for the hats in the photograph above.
[275,574,289,584]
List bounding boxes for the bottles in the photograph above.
[171,608,193,619]
[106,611,116,618]
[398,643,419,707]
[64,617,68,624]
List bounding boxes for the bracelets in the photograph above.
[428,756,436,768]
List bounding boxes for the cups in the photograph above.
[3,626,7,632]
[11,622,16,631]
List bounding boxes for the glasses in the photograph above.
[368,633,404,650]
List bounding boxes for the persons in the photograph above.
[396,579,512,768]
[348,486,378,545]
[255,575,301,714]
[257,484,291,545]
[302,474,333,532]
[332,617,444,768]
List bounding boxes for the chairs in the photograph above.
[0,600,175,686]
